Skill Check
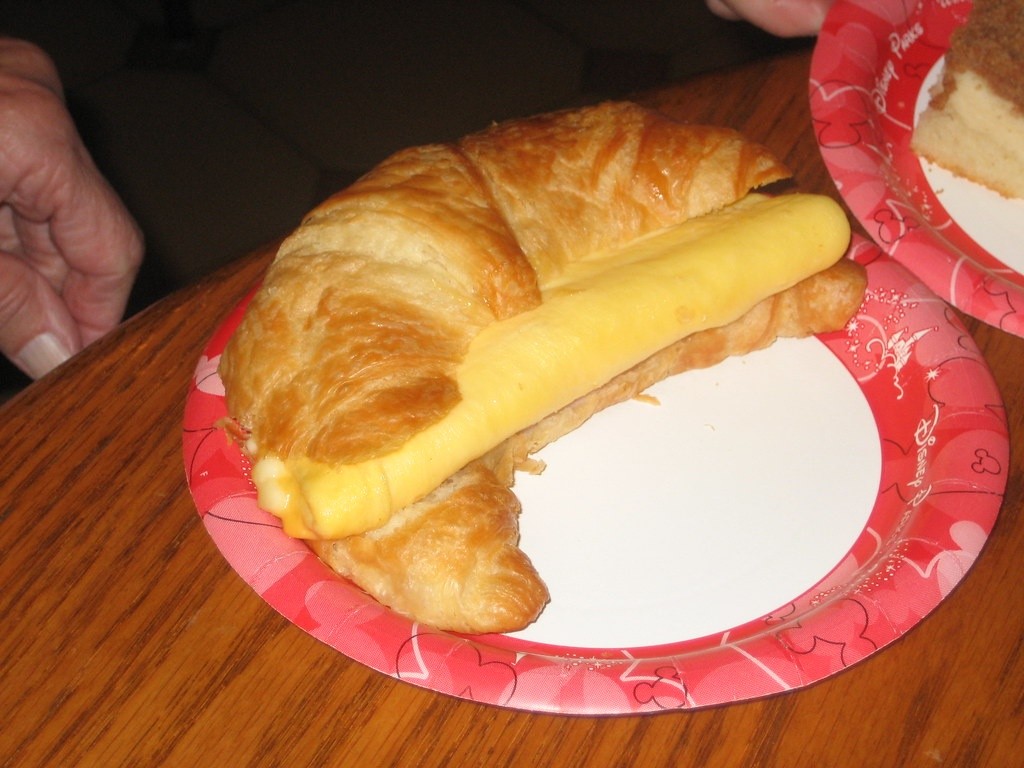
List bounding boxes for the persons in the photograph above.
[0,0,832,381]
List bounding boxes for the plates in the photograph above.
[808,0,1024,340]
[183,235,1009,716]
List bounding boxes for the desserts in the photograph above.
[912,0,1024,204]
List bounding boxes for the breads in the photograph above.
[219,101,867,636]
